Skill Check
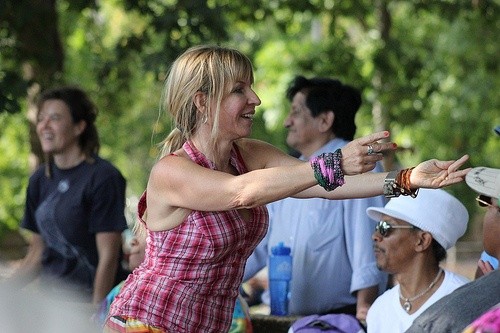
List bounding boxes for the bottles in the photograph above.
[269,242,292,316]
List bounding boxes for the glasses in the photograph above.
[475,195,500,212]
[375,220,416,237]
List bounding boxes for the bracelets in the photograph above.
[310,148,345,192]
[383,166,419,198]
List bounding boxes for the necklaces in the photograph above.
[397,268,444,313]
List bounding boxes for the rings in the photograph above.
[445,176,448,180]
[366,144,373,156]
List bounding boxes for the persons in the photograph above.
[8,87,130,305]
[240,75,390,321]
[460,126,500,333]
[91,218,254,333]
[365,189,474,333]
[101,46,473,333]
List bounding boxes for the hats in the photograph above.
[366,187,469,250]
[465,167,500,199]
